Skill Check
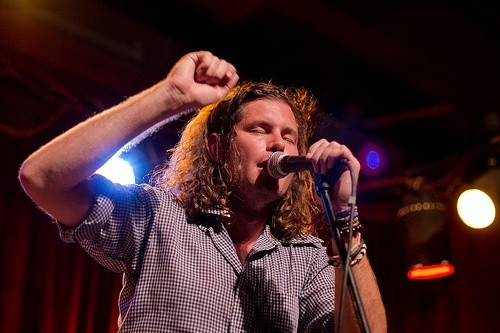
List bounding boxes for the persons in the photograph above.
[19,49,388,333]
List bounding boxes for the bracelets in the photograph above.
[320,208,366,269]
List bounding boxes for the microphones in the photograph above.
[266,151,347,180]
